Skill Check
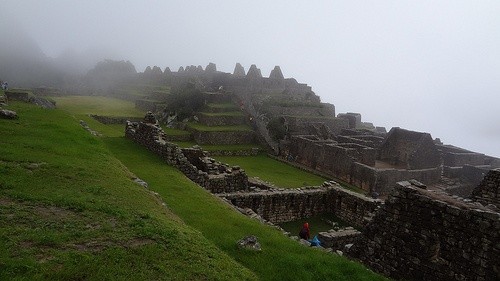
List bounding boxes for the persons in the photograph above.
[303,221,311,241]
